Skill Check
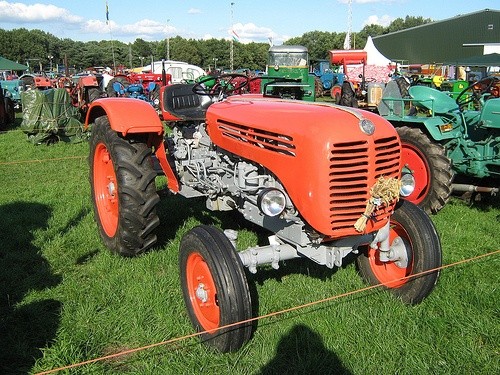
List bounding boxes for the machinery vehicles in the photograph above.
[0,38,172,121]
[81,55,443,353]
[257,47,500,215]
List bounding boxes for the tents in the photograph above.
[0,55,29,80]
[334,36,401,83]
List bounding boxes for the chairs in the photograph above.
[409,85,459,115]
[160,83,211,122]
